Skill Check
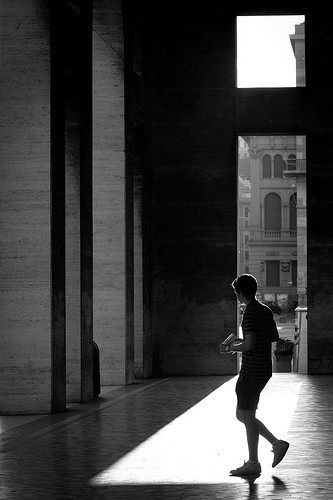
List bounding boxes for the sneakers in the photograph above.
[230,460,261,475]
[271,440,289,468]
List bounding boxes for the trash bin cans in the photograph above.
[93,340,102,397]
[273,337,294,373]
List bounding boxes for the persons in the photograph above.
[229,273,290,476]
